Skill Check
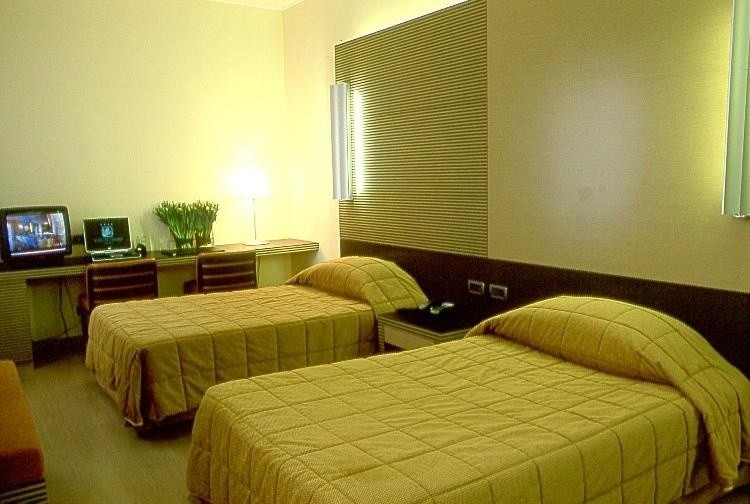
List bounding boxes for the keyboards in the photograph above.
[92,254,140,262]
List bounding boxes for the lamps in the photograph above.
[330,84,352,202]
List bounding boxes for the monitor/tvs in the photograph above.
[0,205,72,269]
[82,217,133,254]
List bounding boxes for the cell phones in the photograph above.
[108,253,123,258]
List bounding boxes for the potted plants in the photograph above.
[195,199,221,248]
[154,200,193,252]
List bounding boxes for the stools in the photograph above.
[0,359,48,504]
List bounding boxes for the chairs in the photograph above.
[183,251,258,294]
[76,257,158,353]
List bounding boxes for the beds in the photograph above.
[185,292,750,504]
[83,255,429,438]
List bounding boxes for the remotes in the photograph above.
[430,305,449,314]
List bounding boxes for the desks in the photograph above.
[0,237,319,364]
[375,306,477,352]
